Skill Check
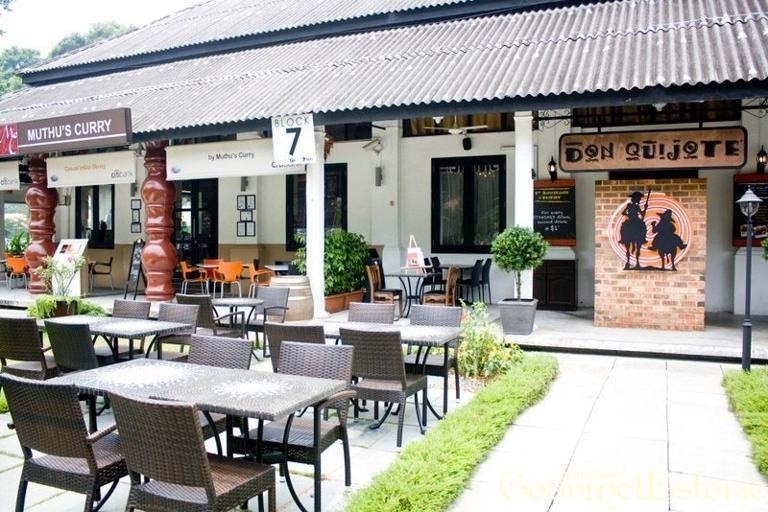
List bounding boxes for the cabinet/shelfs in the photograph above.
[533,259,578,310]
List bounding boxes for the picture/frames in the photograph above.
[246,222,255,236]
[131,198,140,209]
[132,211,140,222]
[246,195,256,209]
[129,223,142,235]
[238,222,246,235]
[241,210,252,221]
[238,196,246,210]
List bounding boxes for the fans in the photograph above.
[424,116,487,141]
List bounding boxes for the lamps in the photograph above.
[547,155,558,182]
[756,145,768,166]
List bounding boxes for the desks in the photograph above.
[387,268,436,318]
[36,313,193,362]
[45,358,346,512]
[292,318,463,414]
[212,299,264,361]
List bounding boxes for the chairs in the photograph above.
[150,304,200,364]
[45,321,102,372]
[422,258,444,294]
[4,253,13,259]
[0,372,125,512]
[106,389,278,512]
[89,256,115,293]
[411,305,463,404]
[364,264,404,319]
[431,257,448,287]
[458,260,482,304]
[275,260,298,275]
[203,258,223,280]
[232,340,356,487]
[248,286,288,357]
[254,258,260,283]
[214,262,241,298]
[375,260,403,320]
[263,320,328,375]
[248,265,275,299]
[339,328,431,446]
[176,293,245,353]
[185,336,253,440]
[1,319,59,428]
[346,300,396,326]
[424,265,455,306]
[480,259,492,304]
[5,251,31,290]
[181,261,209,296]
[96,299,150,362]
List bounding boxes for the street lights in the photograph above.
[735,184,763,373]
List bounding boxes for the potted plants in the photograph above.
[291,228,368,314]
[32,252,87,318]
[490,225,550,335]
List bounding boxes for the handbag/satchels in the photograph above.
[406,235,428,278]
